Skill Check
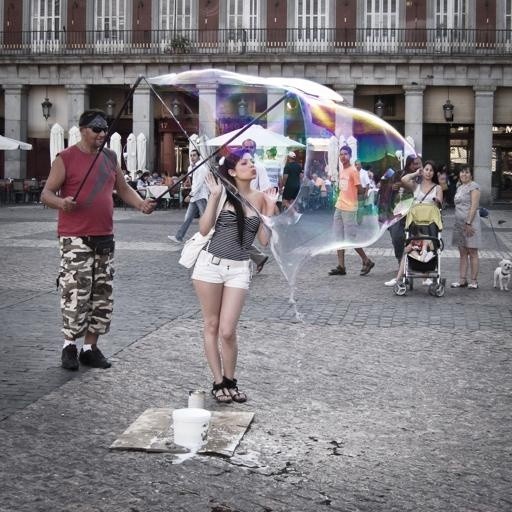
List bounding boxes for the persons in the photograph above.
[282,151,304,225]
[167,150,212,244]
[327,146,374,276]
[383,160,443,286]
[39,109,158,371]
[389,154,424,269]
[449,165,483,289]
[237,139,273,274]
[402,226,433,261]
[181,149,279,403]
[262,146,285,223]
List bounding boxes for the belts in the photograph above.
[199,249,250,270]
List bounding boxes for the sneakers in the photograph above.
[467,278,479,289]
[79,343,112,369]
[294,213,303,225]
[450,277,468,288]
[422,277,433,286]
[167,235,184,244]
[384,277,397,287]
[60,343,79,370]
[287,219,293,226]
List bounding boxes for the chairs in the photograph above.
[0,169,388,212]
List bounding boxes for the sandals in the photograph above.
[224,375,248,403]
[359,258,375,276]
[328,264,347,276]
[211,376,234,404]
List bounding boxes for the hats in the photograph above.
[285,151,297,159]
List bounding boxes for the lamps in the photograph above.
[104,88,117,117]
[238,95,248,118]
[40,88,53,120]
[441,87,456,123]
[373,94,385,120]
[169,94,185,116]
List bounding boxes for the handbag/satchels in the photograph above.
[79,233,116,256]
[176,225,216,271]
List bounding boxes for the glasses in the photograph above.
[83,124,110,134]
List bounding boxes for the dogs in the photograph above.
[493,259,512,291]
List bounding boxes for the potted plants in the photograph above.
[169,35,189,55]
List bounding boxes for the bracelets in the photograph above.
[465,221,471,225]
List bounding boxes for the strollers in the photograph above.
[394,196,449,298]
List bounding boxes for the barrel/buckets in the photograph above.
[170,407,212,448]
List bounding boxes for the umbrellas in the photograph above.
[1,134,34,154]
